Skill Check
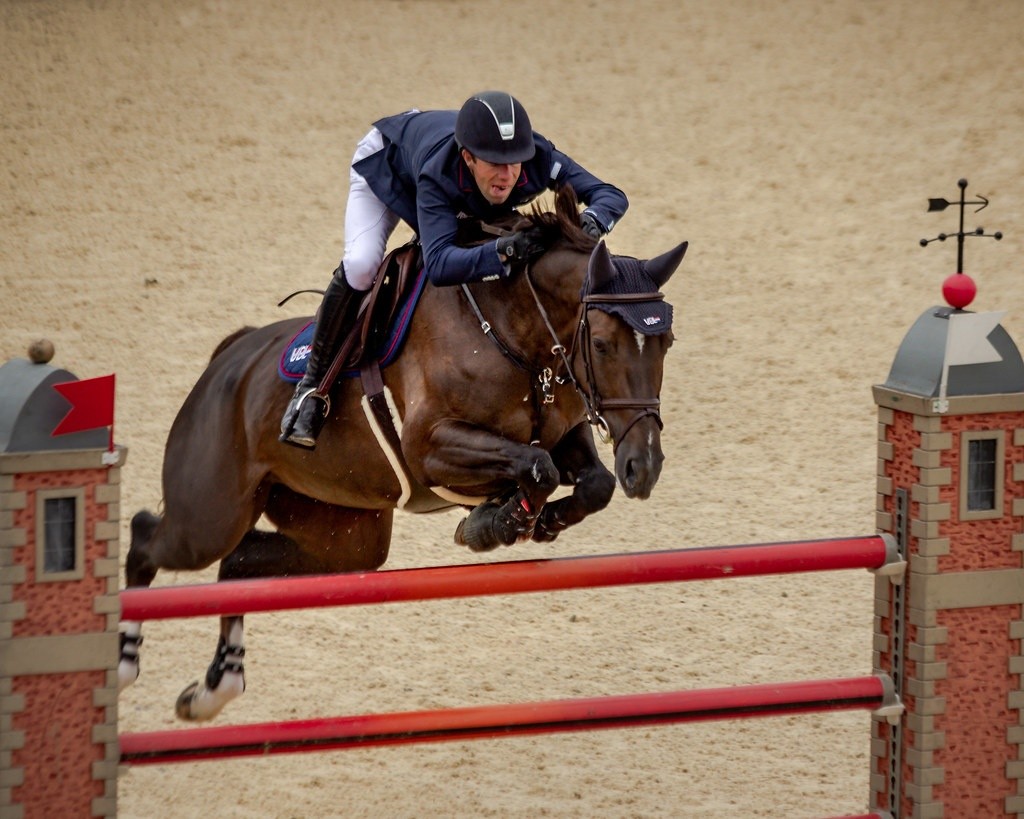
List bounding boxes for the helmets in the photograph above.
[457,91,536,164]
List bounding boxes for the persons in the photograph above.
[278,91,629,449]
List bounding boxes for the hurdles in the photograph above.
[2,273,1024,819]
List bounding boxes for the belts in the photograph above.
[382,109,411,149]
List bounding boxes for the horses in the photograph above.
[117,181,689,723]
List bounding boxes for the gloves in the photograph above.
[497,228,545,262]
[577,212,603,242]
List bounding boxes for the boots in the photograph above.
[280,260,375,446]
